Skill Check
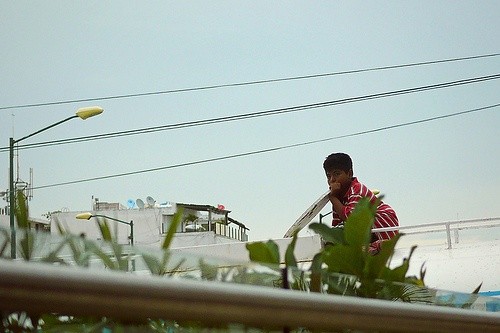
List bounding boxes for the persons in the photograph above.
[323,153,399,256]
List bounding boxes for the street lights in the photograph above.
[9,106,105,260]
[76,212,134,249]
